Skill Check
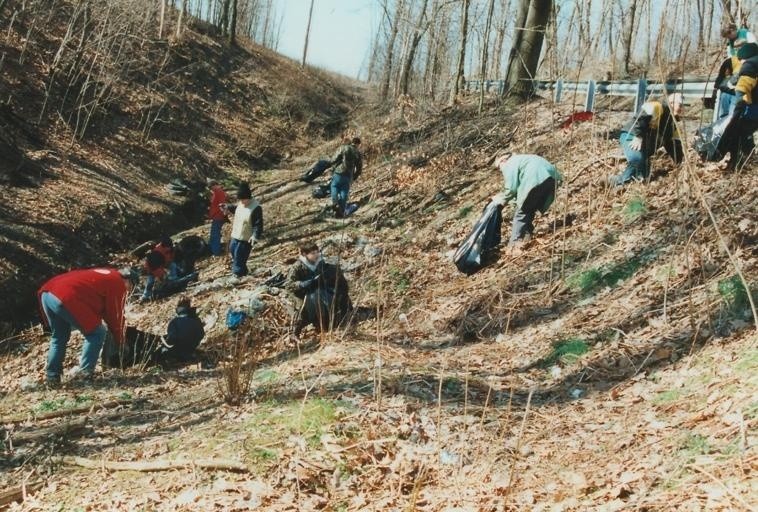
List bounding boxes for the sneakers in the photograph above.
[46,375,94,393]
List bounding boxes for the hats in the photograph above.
[236,180,252,199]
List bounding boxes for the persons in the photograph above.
[36,266,141,385]
[131,178,265,304]
[709,22,758,172]
[617,92,686,184]
[327,136,363,218]
[495,148,564,245]
[283,242,325,343]
